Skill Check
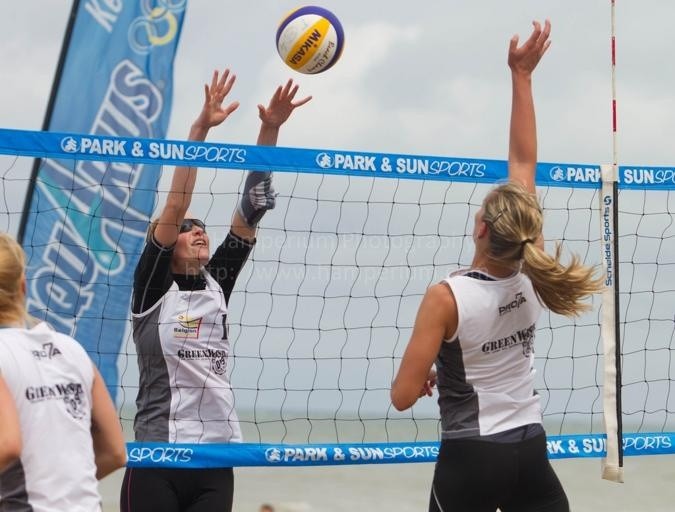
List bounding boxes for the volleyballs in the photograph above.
[275,7,344,74]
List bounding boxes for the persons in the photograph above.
[1,229,128,510]
[118,69,313,511]
[389,20,610,512]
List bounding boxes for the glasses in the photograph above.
[177,219,206,234]
